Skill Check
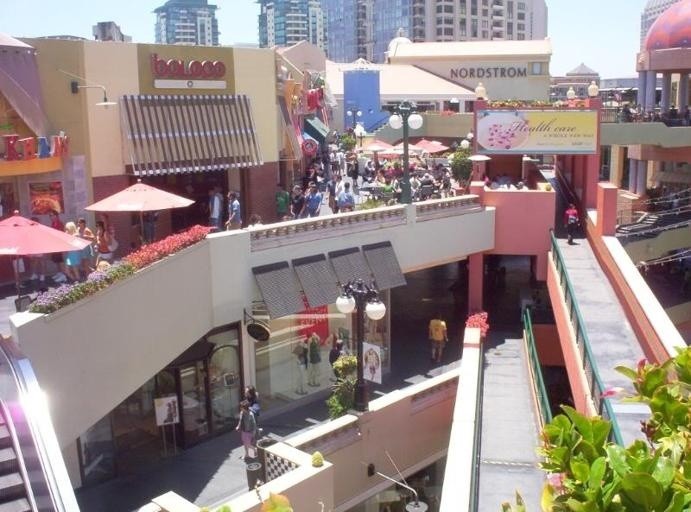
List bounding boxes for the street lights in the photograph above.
[336,275,390,416]
[347,103,364,148]
[388,99,425,202]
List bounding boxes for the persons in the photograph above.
[93,220,114,269]
[234,399,260,461]
[245,384,261,440]
[8,255,29,291]
[324,326,344,381]
[26,215,46,282]
[273,148,452,224]
[308,332,322,387]
[291,338,308,395]
[563,203,581,246]
[621,102,690,122]
[76,216,95,275]
[207,187,224,229]
[339,321,353,354]
[226,191,243,233]
[248,213,264,229]
[427,311,450,364]
[48,209,68,283]
[364,348,380,382]
[64,221,87,282]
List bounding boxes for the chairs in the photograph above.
[362,181,453,201]
[618,107,691,126]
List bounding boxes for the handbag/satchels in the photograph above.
[107,237,118,252]
[569,216,576,225]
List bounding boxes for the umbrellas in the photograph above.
[378,142,418,156]
[417,140,450,155]
[395,141,425,152]
[0,208,95,312]
[85,177,198,245]
[356,138,395,152]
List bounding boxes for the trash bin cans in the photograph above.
[256,439,279,475]
